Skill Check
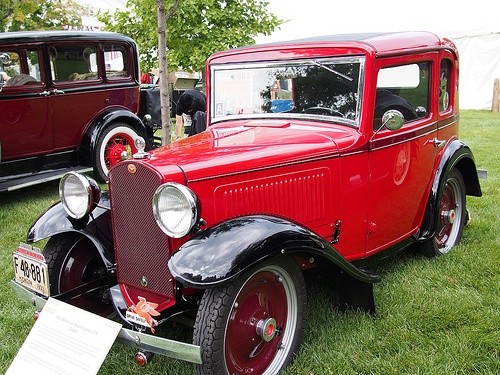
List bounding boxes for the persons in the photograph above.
[174,89,207,137]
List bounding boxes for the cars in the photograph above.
[11,33,482,375]
[0,28,147,201]
